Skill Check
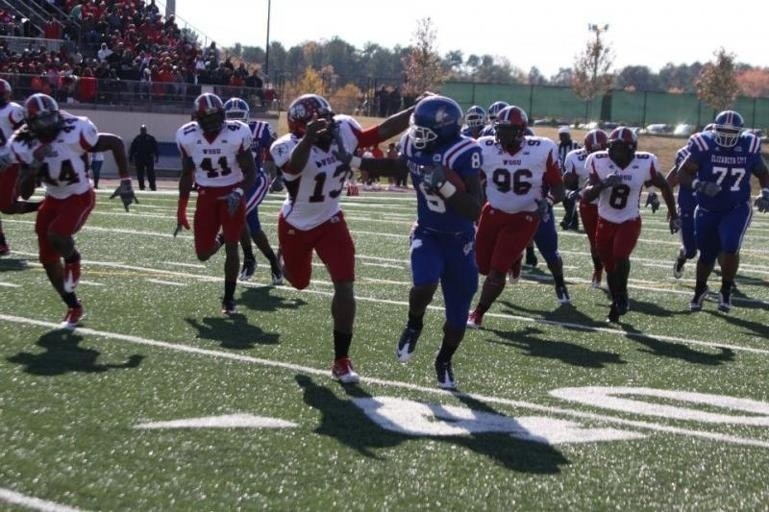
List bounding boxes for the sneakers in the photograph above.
[330,358,361,384]
[688,285,709,311]
[591,265,604,288]
[0,233,9,255]
[560,215,578,231]
[555,285,572,304]
[508,254,524,284]
[222,301,236,319]
[62,249,82,294]
[465,311,483,329]
[63,298,85,327]
[276,244,286,270]
[605,299,631,328]
[673,248,688,279]
[718,290,732,313]
[432,360,459,391]
[396,327,422,362]
[270,263,285,285]
[520,257,537,274]
[240,256,257,281]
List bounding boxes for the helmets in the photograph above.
[558,127,571,140]
[713,109,745,148]
[584,129,608,154]
[23,92,60,121]
[609,126,637,154]
[465,100,529,145]
[407,94,464,152]
[0,79,13,93]
[189,92,250,123]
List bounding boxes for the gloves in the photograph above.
[754,191,769,213]
[600,173,622,189]
[418,162,446,195]
[563,172,579,191]
[530,197,550,220]
[109,176,140,213]
[216,187,245,215]
[578,183,595,200]
[645,192,660,213]
[669,215,683,235]
[33,144,52,163]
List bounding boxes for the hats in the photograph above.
[286,93,336,130]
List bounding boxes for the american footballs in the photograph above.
[436,168,467,198]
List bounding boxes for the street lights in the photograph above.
[587,22,609,122]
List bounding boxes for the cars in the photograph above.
[534,117,698,137]
[738,128,767,141]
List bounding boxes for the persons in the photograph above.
[269,92,414,381]
[1,1,280,113]
[173,86,424,317]
[1,79,160,322]
[460,102,769,322]
[334,97,487,389]
